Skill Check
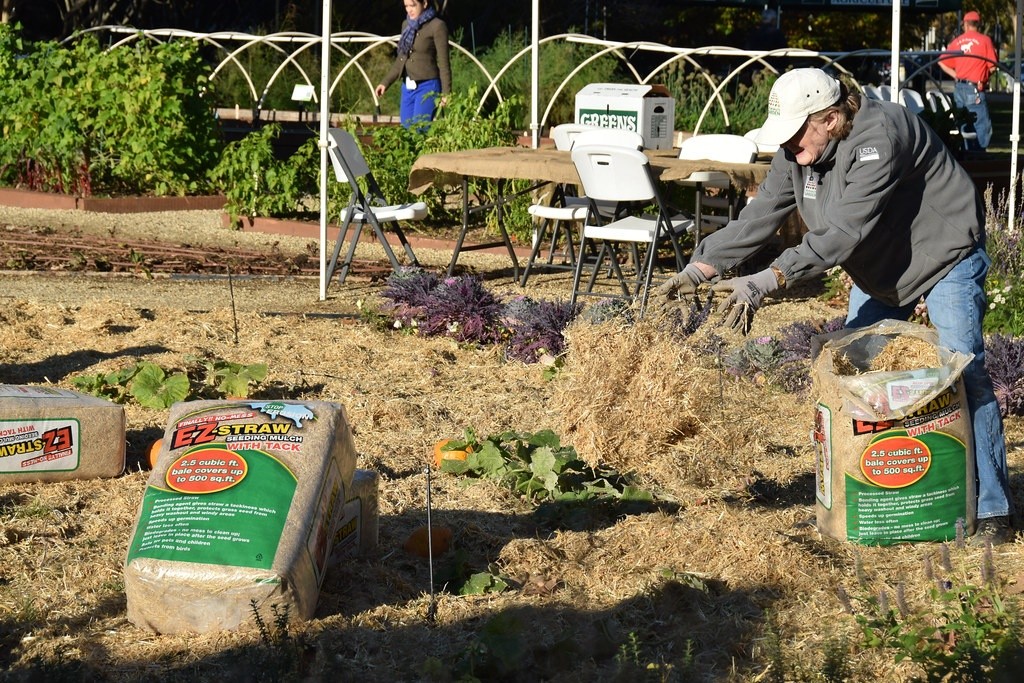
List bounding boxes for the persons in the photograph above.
[652,70,1011,552]
[939,11,997,181]
[374,0,453,137]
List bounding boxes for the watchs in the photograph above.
[772,268,786,288]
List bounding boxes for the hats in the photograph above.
[756,68,843,147]
[961,11,980,24]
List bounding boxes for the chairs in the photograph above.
[318,128,430,294]
[642,131,760,315]
[520,122,621,297]
[568,144,704,328]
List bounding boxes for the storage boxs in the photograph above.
[572,81,677,150]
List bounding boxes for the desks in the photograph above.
[404,148,774,287]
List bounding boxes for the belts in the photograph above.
[954,79,989,88]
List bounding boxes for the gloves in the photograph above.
[652,266,706,314]
[711,269,777,333]
